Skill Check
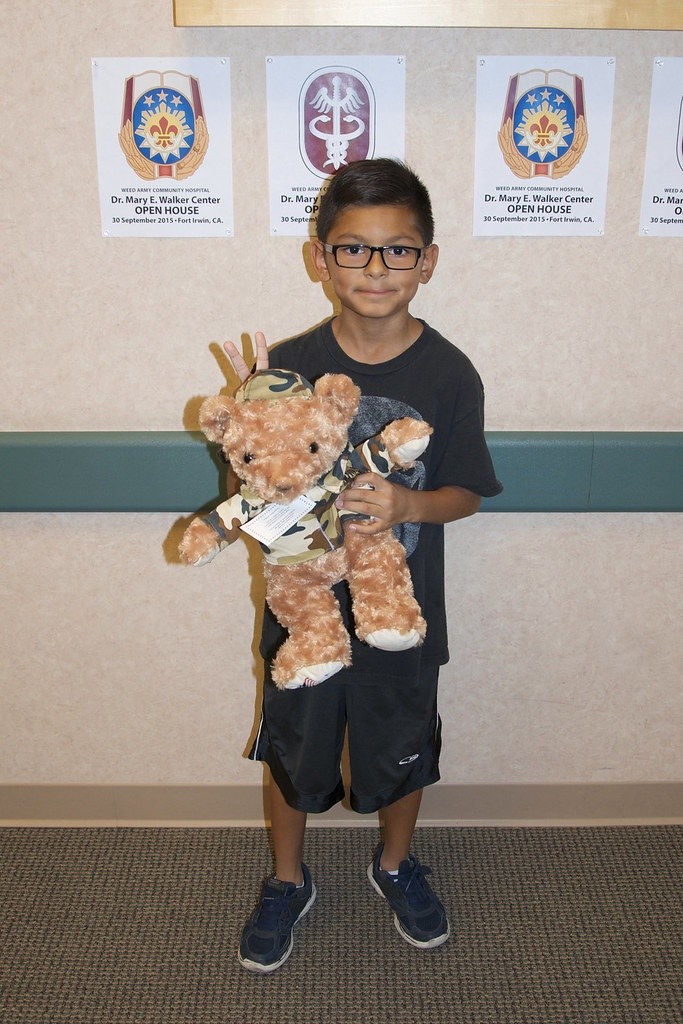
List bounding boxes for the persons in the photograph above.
[223,157,504,968]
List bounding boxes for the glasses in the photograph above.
[315,240,433,271]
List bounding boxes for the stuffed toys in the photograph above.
[179,369,433,688]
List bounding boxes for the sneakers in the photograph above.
[366,842,451,950]
[236,860,318,973]
